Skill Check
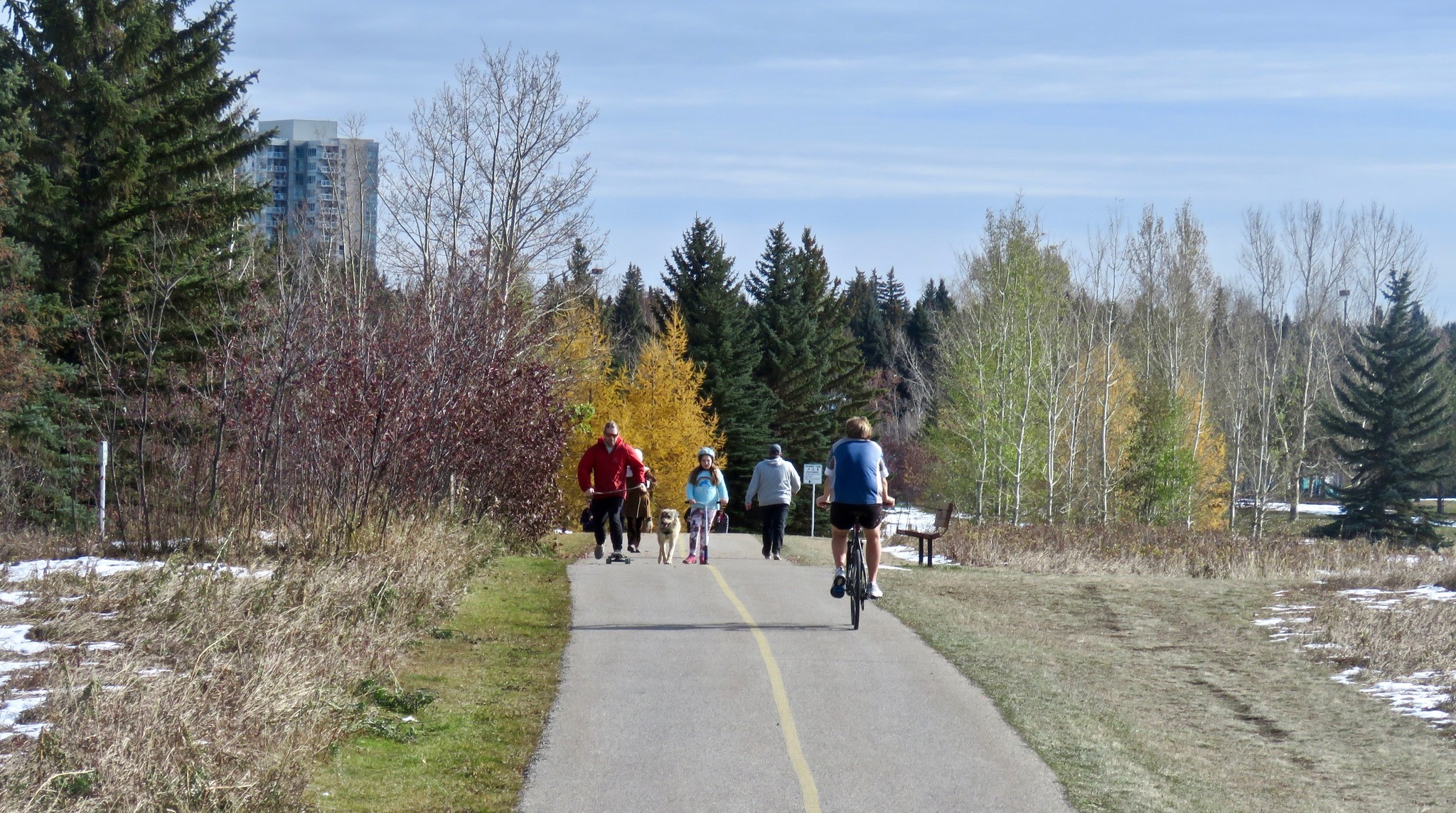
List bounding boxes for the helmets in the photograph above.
[697,448,715,459]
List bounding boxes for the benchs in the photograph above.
[896,502,953,567]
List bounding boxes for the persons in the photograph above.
[682,448,729,564]
[714,507,729,533]
[745,444,801,561]
[579,500,593,533]
[577,419,647,559]
[625,449,656,553]
[816,415,896,599]
[684,506,691,533]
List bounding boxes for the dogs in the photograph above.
[658,509,681,565]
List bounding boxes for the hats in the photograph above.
[770,444,781,456]
[634,449,643,462]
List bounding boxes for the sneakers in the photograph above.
[699,556,705,564]
[866,582,882,599]
[831,568,846,598]
[683,554,697,564]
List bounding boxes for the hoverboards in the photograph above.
[685,500,722,565]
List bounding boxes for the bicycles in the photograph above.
[822,501,890,631]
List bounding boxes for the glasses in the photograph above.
[604,431,618,438]
[719,511,723,512]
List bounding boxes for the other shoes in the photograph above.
[628,544,636,553]
[762,547,770,559]
[773,552,780,561]
[631,549,640,553]
[595,540,606,559]
[611,550,623,557]
